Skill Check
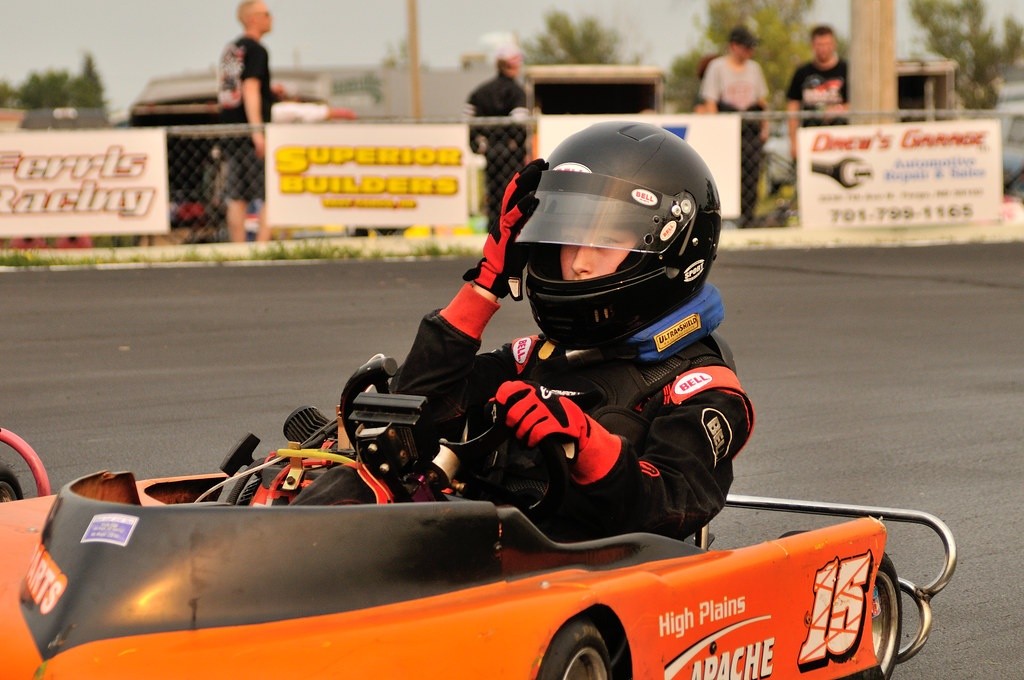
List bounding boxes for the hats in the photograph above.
[498,44,524,66]
[730,28,765,47]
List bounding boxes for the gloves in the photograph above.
[461,158,543,299]
[481,380,623,486]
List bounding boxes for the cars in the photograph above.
[18,66,357,242]
[751,70,1024,205]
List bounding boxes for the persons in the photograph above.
[216,0,285,241]
[698,25,770,229]
[393,121,755,540]
[463,45,529,233]
[786,24,849,162]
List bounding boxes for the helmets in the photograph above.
[526,119,723,351]
